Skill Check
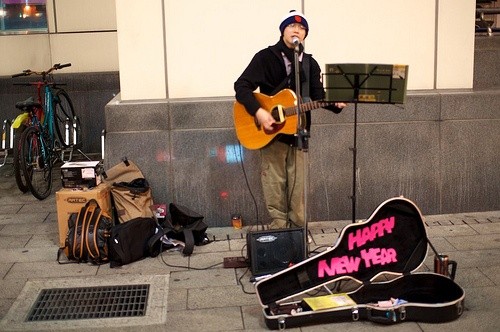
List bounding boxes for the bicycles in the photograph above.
[11,63,83,200]
[10,81,68,193]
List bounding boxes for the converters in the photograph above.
[223,257,247,269]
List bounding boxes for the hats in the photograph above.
[279,9,308,33]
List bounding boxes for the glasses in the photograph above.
[287,24,306,29]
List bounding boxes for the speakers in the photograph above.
[246,227,305,276]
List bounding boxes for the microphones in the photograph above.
[293,36,299,46]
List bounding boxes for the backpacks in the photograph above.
[57,158,208,268]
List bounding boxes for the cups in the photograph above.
[231,214,243,229]
[434,253,457,279]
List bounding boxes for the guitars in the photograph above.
[233,89,335,150]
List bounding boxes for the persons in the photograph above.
[234,10,346,230]
